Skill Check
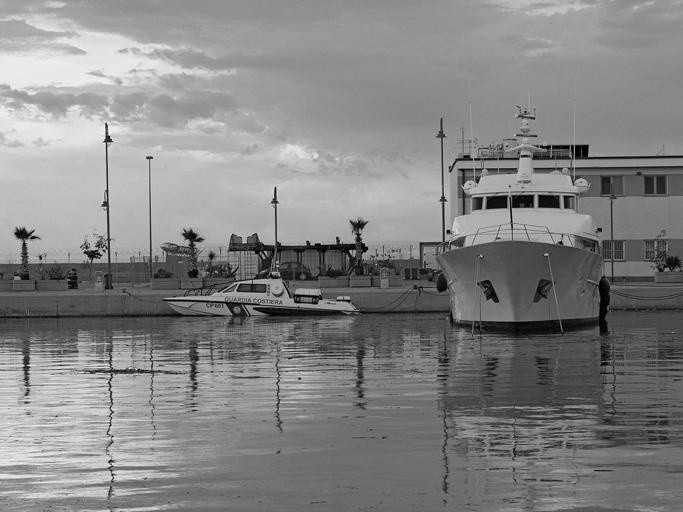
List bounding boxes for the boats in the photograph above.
[162,277,360,317]
[433,88,605,328]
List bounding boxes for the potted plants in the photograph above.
[11,225,72,291]
[151,228,239,290]
[646,229,683,283]
[317,217,406,287]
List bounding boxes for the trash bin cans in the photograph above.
[405,268,418,280]
[104,274,113,289]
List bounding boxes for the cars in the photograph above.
[259,262,312,280]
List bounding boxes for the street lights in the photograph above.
[435,118,448,252]
[608,184,617,282]
[101,122,113,289]
[146,155,154,276]
[270,186,280,272]
